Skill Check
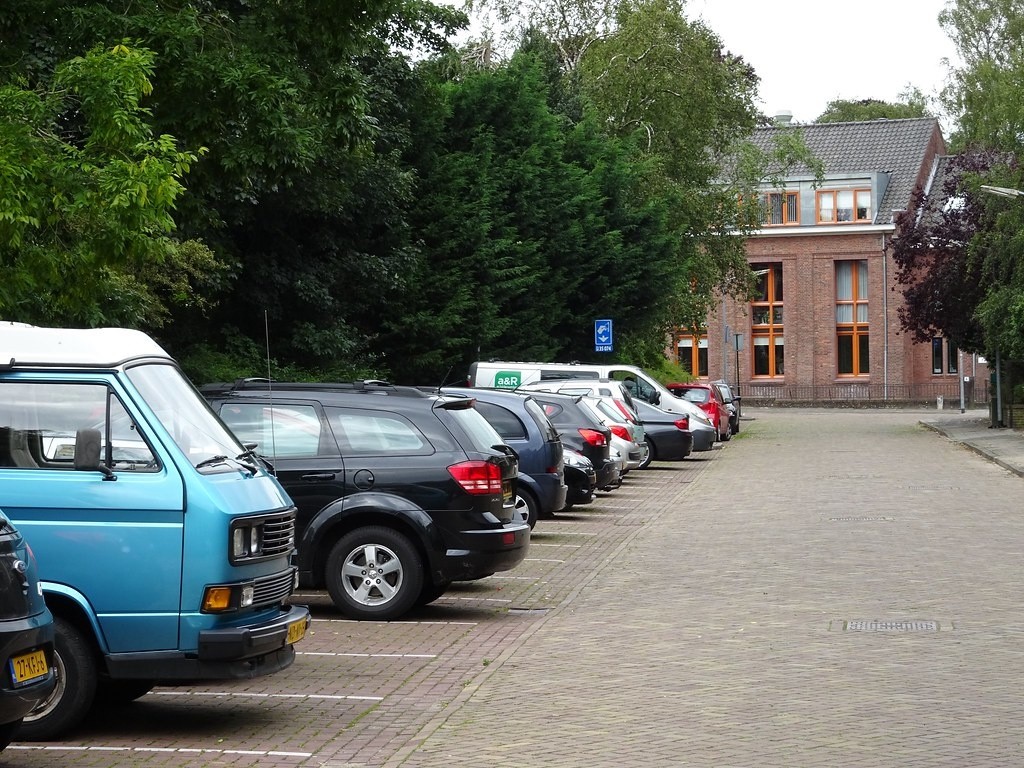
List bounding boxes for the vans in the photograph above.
[0,319,311,743]
[468,359,717,452]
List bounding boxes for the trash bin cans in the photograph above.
[937,394,943,411]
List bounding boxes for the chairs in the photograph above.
[1,408,40,467]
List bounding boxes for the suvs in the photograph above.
[342,382,568,532]
[71,377,532,622]
[477,377,694,493]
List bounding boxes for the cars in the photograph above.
[0,508,57,752]
[493,419,597,513]
[713,382,742,433]
[659,381,734,443]
